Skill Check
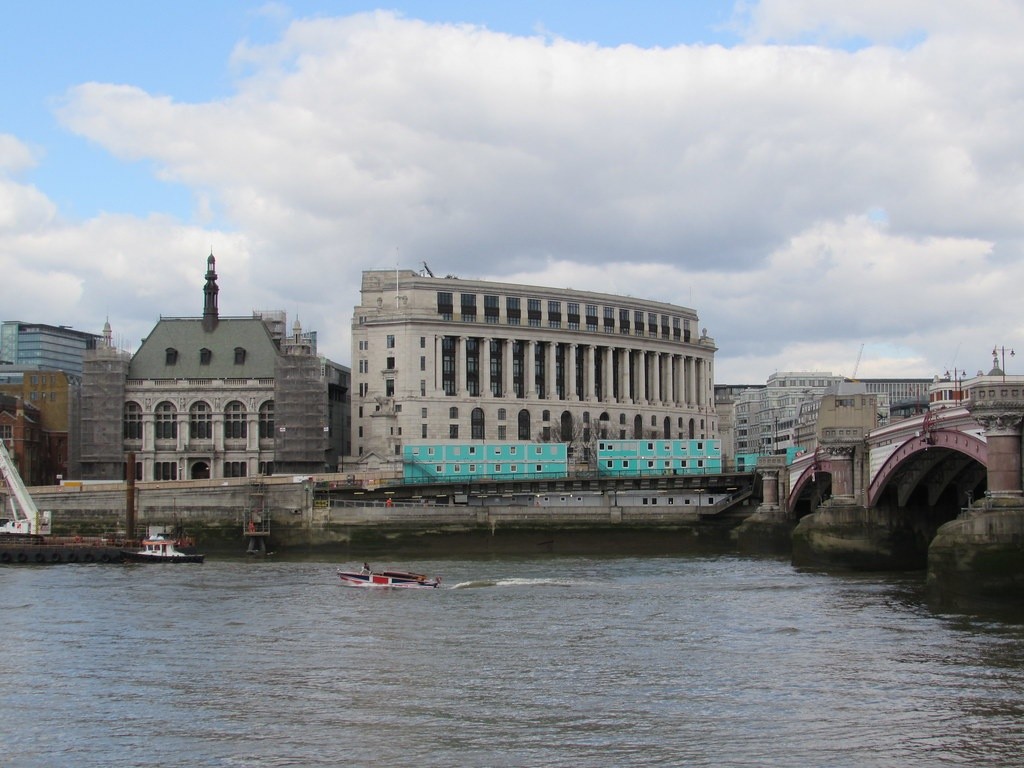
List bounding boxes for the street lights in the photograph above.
[908,384,928,415]
[991,344,1016,380]
[944,367,968,407]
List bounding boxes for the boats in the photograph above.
[336,569,442,589]
[118,535,206,563]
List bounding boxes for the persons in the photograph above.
[386,497,392,507]
[364,562,370,571]
